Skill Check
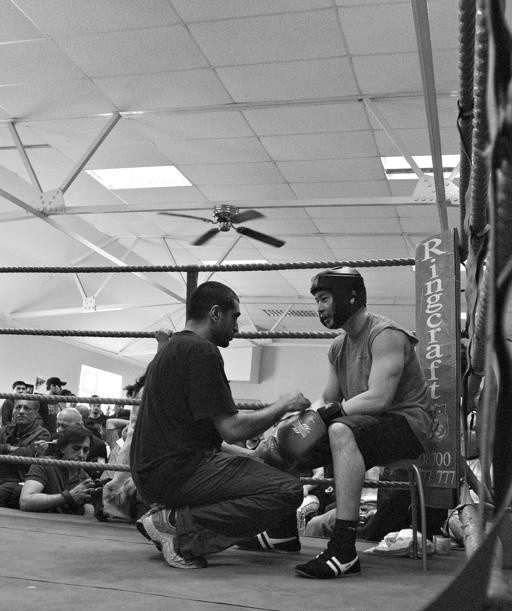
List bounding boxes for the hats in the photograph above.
[46,378,66,385]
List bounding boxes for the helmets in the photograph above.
[311,267,366,328]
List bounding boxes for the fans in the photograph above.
[159,202,285,249]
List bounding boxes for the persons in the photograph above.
[221,407,409,543]
[235,265,438,581]
[2,376,165,522]
[128,280,311,571]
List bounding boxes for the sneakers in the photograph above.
[136,506,207,568]
[296,549,361,579]
[235,531,301,553]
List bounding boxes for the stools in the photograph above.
[373,457,428,571]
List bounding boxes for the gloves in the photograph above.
[258,437,298,472]
[272,402,346,458]
